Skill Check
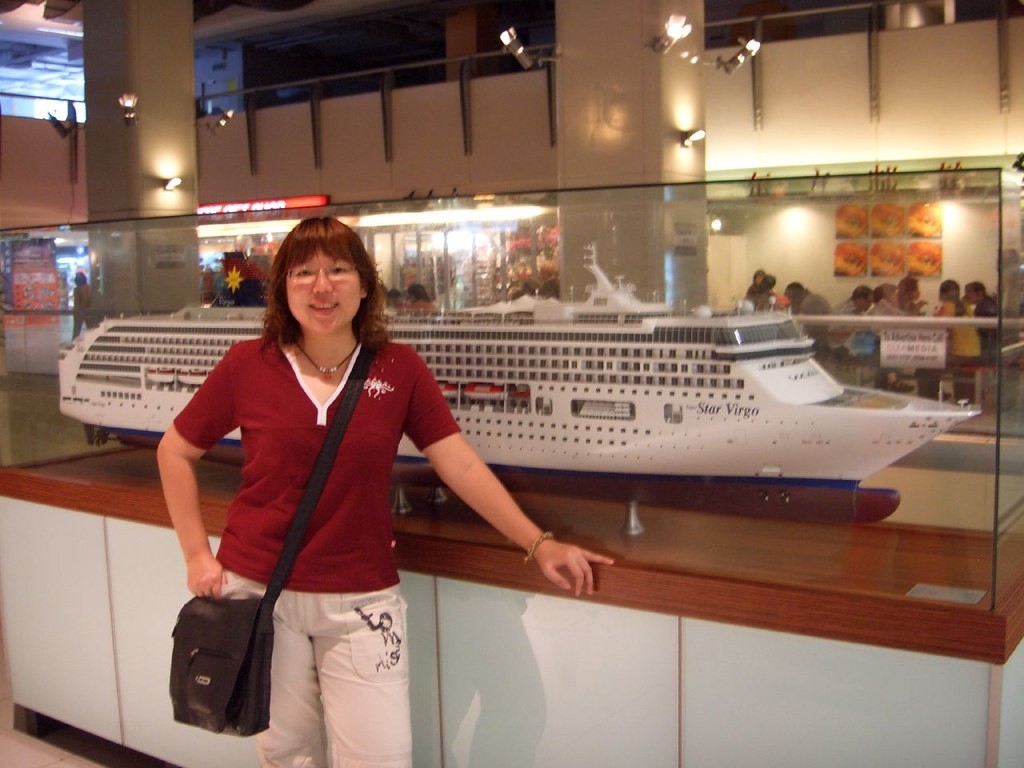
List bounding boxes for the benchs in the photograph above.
[946,339,1024,408]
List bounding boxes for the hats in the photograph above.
[747,270,776,295]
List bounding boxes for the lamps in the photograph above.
[165,178,182,191]
[682,125,706,146]
[46,98,88,137]
[716,38,761,75]
[652,14,691,57]
[114,92,139,125]
[197,107,236,136]
[501,26,559,71]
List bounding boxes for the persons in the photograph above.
[72,271,95,337]
[388,281,439,311]
[744,271,998,406]
[155,216,610,768]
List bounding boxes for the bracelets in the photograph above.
[524,532,553,564]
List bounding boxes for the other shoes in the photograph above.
[875,379,882,388]
[893,380,914,392]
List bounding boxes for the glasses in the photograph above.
[287,263,357,283]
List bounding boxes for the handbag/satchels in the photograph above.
[169,597,274,736]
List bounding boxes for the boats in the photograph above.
[146,372,207,385]
[434,383,529,402]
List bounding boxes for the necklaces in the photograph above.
[295,340,360,380]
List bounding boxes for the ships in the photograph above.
[58,243,984,489]
